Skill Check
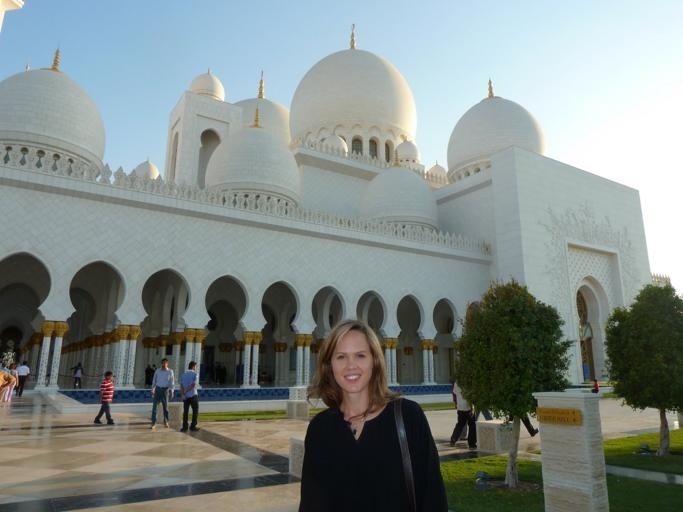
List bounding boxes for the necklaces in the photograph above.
[345,413,365,435]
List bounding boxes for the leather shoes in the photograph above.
[151,423,156,429]
[180,429,187,432]
[164,422,169,427]
[191,427,200,431]
[531,428,539,437]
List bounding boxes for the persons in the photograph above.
[178,361,201,432]
[506,412,540,436]
[297,318,450,511]
[149,359,175,430]
[69,361,83,389]
[0,360,30,401]
[93,370,115,425]
[590,379,599,393]
[200,360,243,385]
[473,404,495,421]
[458,420,469,441]
[144,364,156,390]
[450,379,478,448]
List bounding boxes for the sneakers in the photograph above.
[94,420,102,424]
[468,445,477,450]
[107,422,115,424]
